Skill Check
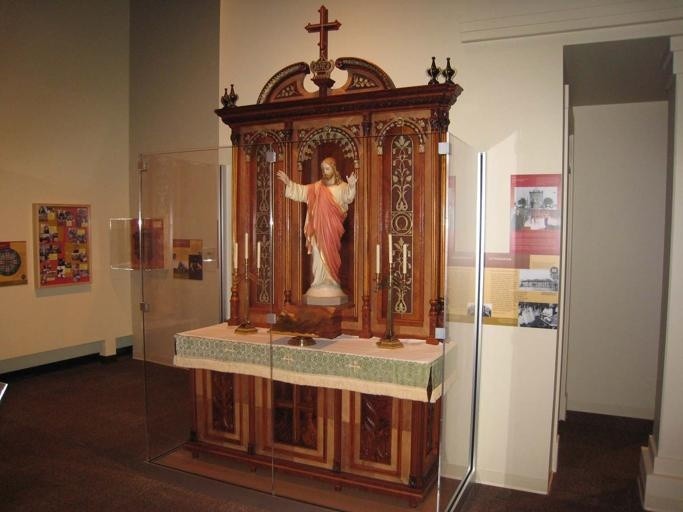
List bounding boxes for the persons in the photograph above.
[276,157,358,286]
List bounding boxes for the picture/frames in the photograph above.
[32,202,93,288]
